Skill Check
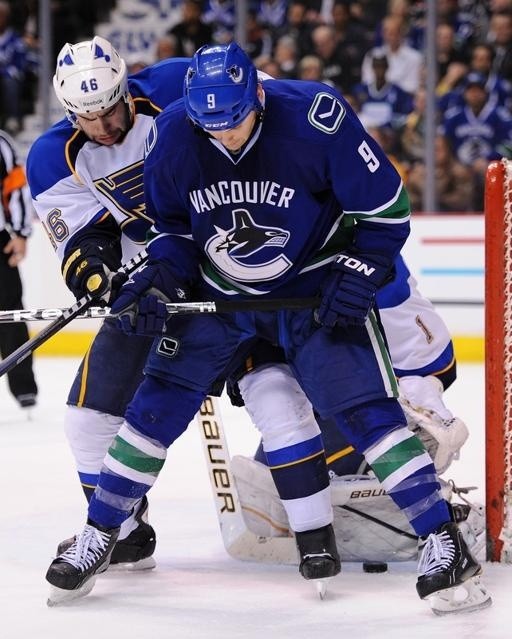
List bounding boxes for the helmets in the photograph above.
[52,36,129,130]
[184,43,257,134]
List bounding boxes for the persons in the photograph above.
[0,130,37,405]
[26,34,341,581]
[47,40,484,599]
[228,252,487,566]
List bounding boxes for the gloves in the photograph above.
[111,263,186,343]
[313,252,394,328]
[61,243,128,309]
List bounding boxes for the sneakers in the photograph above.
[15,394,36,406]
[295,524,341,579]
[413,522,483,600]
[47,522,121,590]
[56,522,156,567]
[457,502,486,538]
